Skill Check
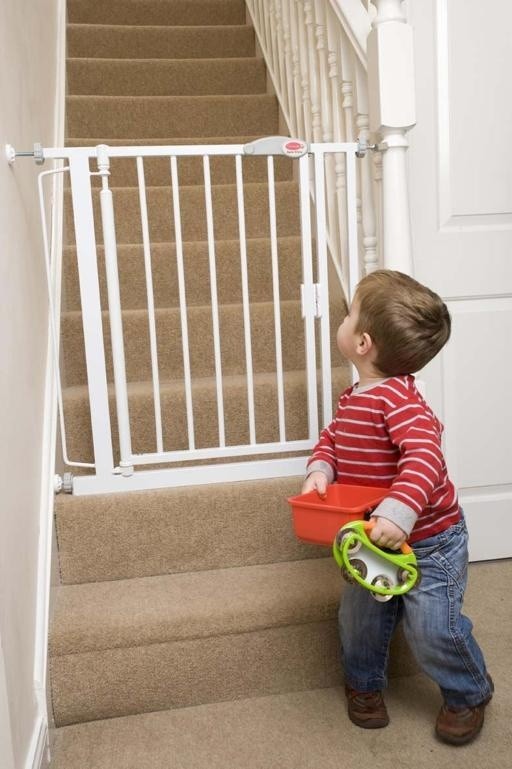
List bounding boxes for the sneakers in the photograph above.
[435,684,494,744]
[344,683,389,728]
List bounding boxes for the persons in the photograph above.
[299,269,496,747]
